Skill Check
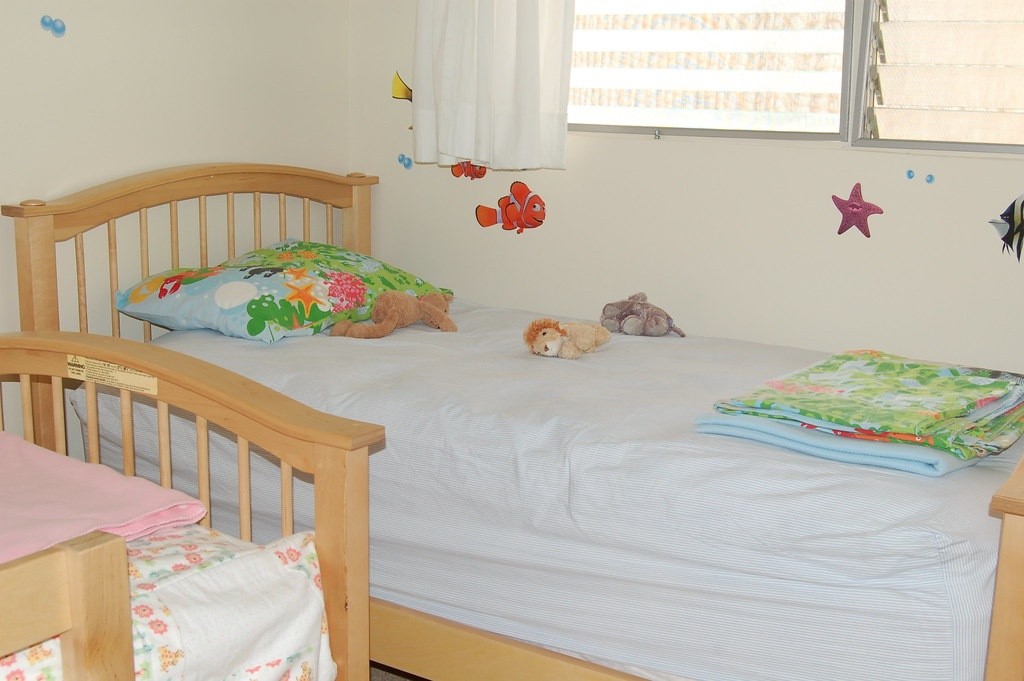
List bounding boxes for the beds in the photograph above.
[0,162,1024,681]
[0,327,386,681]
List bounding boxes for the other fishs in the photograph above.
[989,193,1024,261]
[476,181,545,235]
[451,160,486,180]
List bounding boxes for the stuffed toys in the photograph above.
[601,292,686,337]
[523,319,612,359]
[329,290,457,339]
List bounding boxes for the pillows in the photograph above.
[113,237,452,343]
